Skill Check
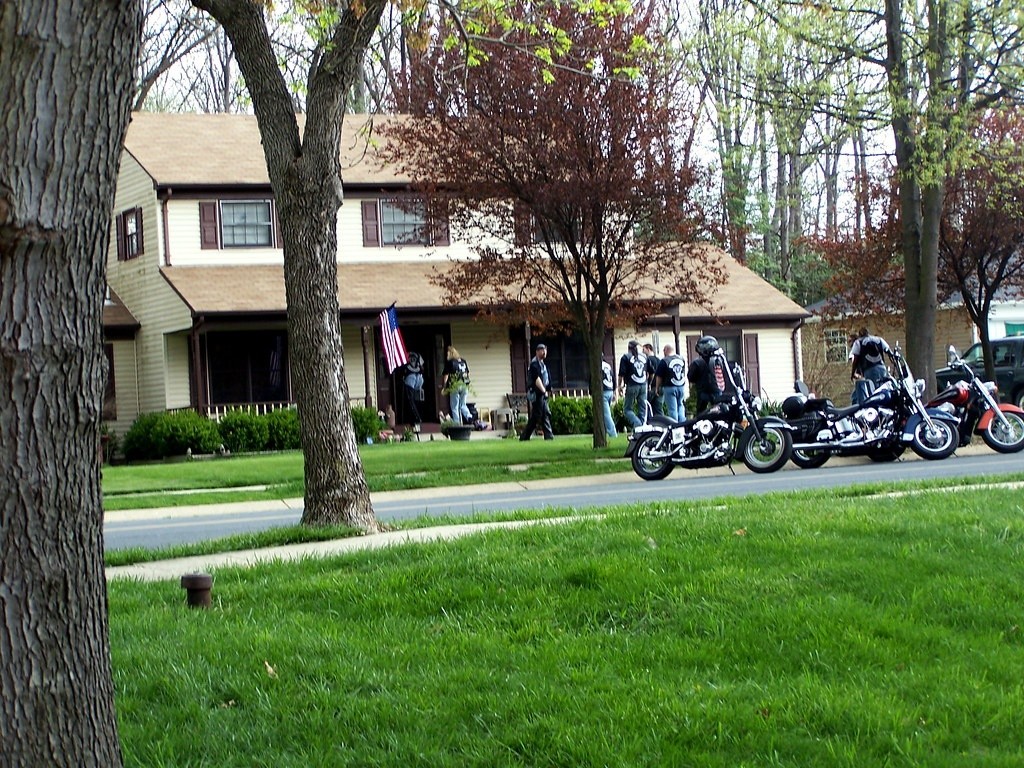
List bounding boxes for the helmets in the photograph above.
[694,335,719,357]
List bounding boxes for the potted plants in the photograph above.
[441,371,476,440]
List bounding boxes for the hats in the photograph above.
[536,344,545,348]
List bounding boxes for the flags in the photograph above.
[379,304,409,373]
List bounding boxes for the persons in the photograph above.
[642,344,665,416]
[589,352,618,437]
[617,341,649,427]
[442,347,477,427]
[656,345,687,423]
[402,345,426,433]
[688,336,727,416]
[521,344,555,442]
[848,327,893,405]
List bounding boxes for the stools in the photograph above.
[478,406,491,423]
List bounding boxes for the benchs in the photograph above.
[507,391,559,422]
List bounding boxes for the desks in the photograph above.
[492,408,515,430]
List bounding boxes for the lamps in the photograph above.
[412,420,421,433]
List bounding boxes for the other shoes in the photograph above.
[412,423,421,433]
[468,418,473,424]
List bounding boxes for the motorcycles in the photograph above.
[865,345,1024,465]
[781,339,962,470]
[623,345,798,481]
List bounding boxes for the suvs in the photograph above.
[935,335,1024,412]
